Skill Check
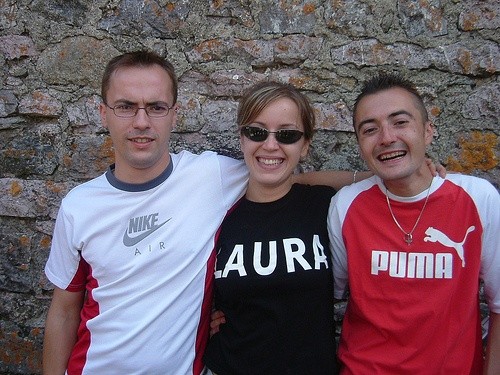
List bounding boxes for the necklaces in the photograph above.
[386,171,437,244]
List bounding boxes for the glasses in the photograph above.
[242,126,305,145]
[104,102,176,118]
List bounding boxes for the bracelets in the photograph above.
[352,169,360,183]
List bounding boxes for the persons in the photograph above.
[41,51,445,375]
[209,73,500,375]
[199,81,339,375]
